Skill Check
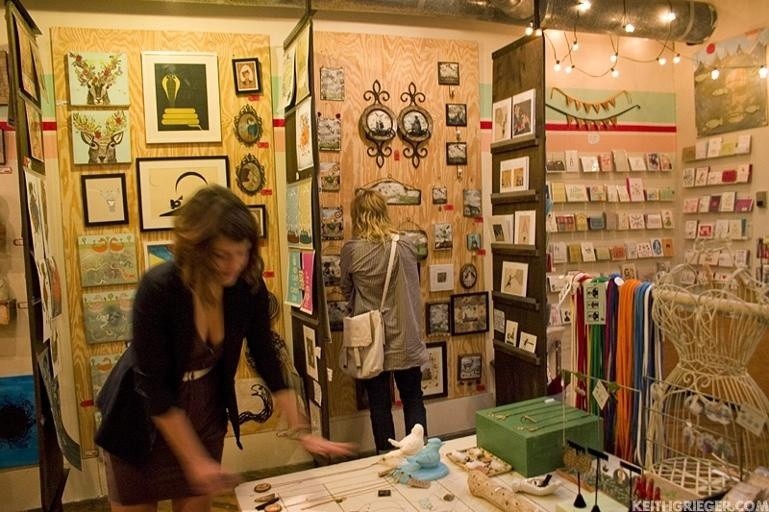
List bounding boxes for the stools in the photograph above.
[183,367,211,383]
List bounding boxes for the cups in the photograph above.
[210,247,250,262]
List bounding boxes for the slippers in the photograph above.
[340,233,399,379]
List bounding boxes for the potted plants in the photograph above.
[284,251,302,308]
[501,260,529,298]
[321,207,344,241]
[360,80,398,168]
[460,264,478,289]
[493,309,505,333]
[234,104,263,146]
[485,214,513,245]
[355,172,421,205]
[457,354,483,379]
[446,142,467,165]
[491,98,512,142]
[144,241,178,274]
[400,82,433,169]
[391,341,448,401]
[294,22,309,105]
[67,53,130,106]
[136,156,231,231]
[24,168,61,344]
[0,374,40,468]
[72,111,132,164]
[452,292,489,334]
[296,96,314,171]
[82,290,135,345]
[286,177,312,249]
[316,111,341,150]
[326,300,351,331]
[247,206,266,238]
[693,26,769,139]
[17,17,40,102]
[319,162,340,191]
[36,349,81,475]
[77,234,138,286]
[463,189,482,217]
[500,156,529,193]
[438,62,460,85]
[278,51,294,107]
[435,224,452,251]
[320,68,345,100]
[24,102,43,162]
[235,153,266,197]
[505,320,519,347]
[230,379,284,433]
[301,324,318,383]
[300,249,315,316]
[140,52,223,145]
[320,255,343,285]
[234,58,259,92]
[514,210,536,245]
[91,356,122,405]
[583,284,607,325]
[512,89,536,138]
[447,104,467,126]
[426,302,449,333]
[518,331,538,353]
[82,174,128,226]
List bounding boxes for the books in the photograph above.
[476,396,604,477]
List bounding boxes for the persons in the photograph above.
[93,183,360,511]
[339,185,429,455]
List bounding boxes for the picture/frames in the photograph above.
[234,432,710,512]
